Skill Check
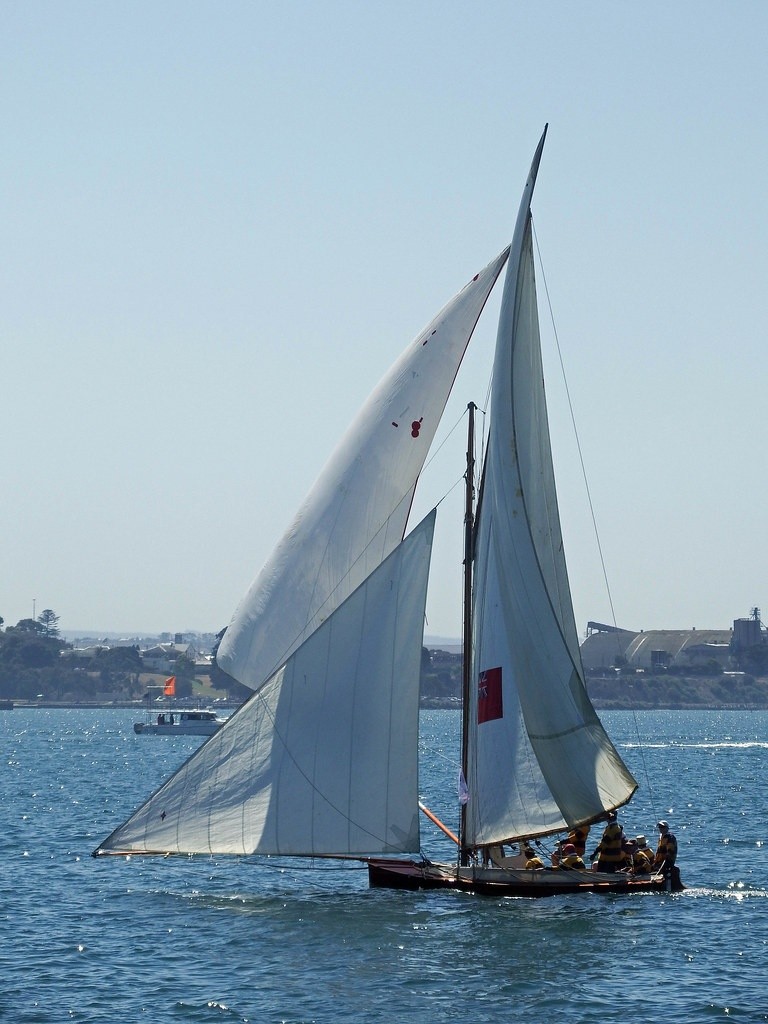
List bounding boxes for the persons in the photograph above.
[158,714,165,725]
[524,848,545,869]
[590,811,677,874]
[545,825,590,871]
[170,714,174,725]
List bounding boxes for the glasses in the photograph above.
[556,843,559,844]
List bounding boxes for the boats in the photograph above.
[134,685,225,736]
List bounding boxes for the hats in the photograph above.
[657,820,669,828]
[636,835,649,845]
[626,839,638,845]
[562,844,574,852]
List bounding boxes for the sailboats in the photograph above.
[91,124,691,892]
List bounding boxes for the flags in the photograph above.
[477,667,504,724]
[164,676,175,696]
